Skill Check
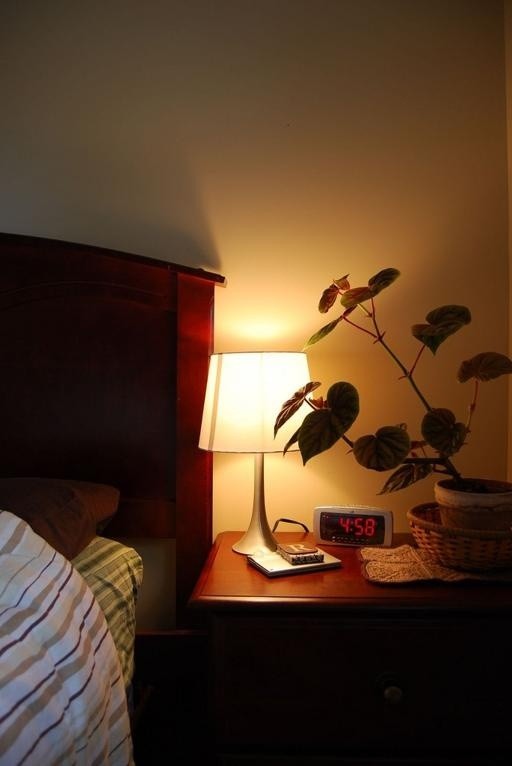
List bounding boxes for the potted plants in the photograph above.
[276,262,511,533]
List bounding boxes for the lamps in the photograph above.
[195,349,317,557]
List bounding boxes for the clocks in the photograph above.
[309,506,396,548]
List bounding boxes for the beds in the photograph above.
[0,228,225,766]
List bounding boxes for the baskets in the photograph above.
[406,502,512,573]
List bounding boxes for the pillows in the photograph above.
[1,478,123,558]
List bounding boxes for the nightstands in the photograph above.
[188,527,511,763]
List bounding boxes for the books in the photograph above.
[246,546,343,579]
[276,542,325,566]
